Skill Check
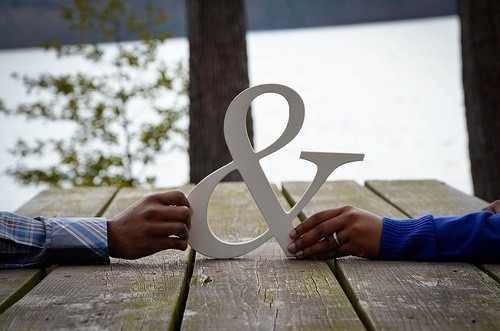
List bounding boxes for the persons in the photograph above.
[287,200,500,265]
[0,190,193,269]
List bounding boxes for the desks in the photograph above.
[0,180,500,331]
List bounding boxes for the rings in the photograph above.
[333,232,341,245]
